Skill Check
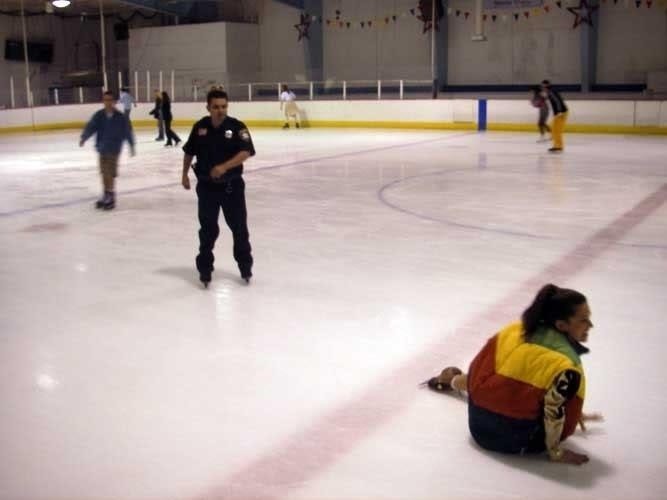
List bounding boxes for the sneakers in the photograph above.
[96,191,116,208]
[239,268,252,278]
[200,269,211,283]
[437,367,463,391]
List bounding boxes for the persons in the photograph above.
[118,87,138,120]
[182,90,256,282]
[79,91,137,208]
[279,84,300,129]
[149,88,182,147]
[529,79,569,151]
[429,283,594,465]
